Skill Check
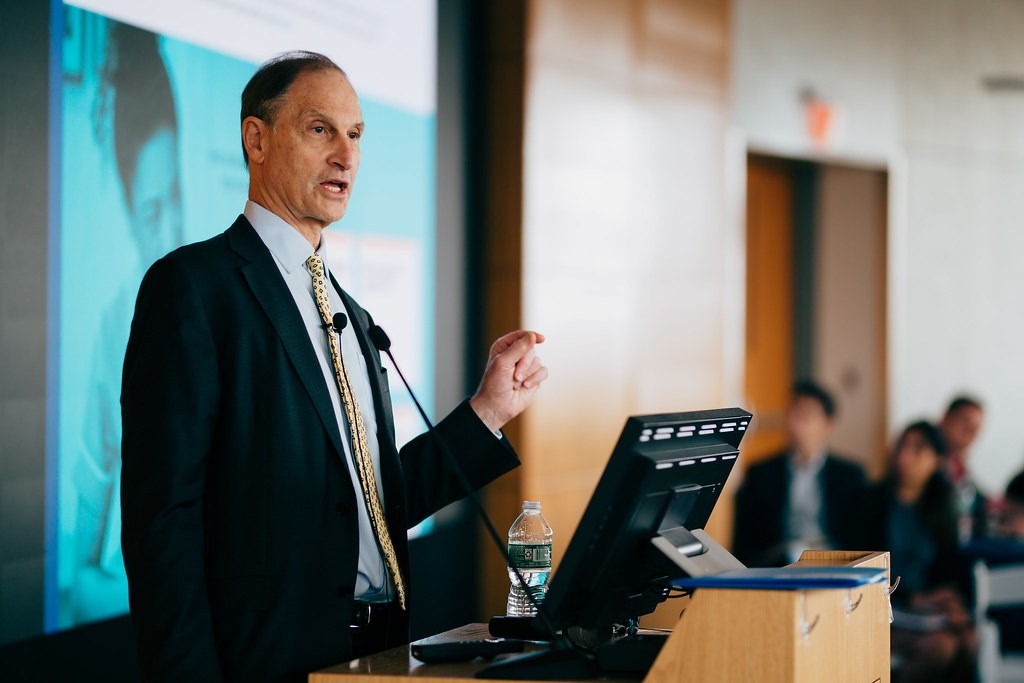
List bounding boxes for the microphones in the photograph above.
[366,325,602,678]
[487,615,601,646]
[334,312,348,336]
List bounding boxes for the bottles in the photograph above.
[507,500,553,617]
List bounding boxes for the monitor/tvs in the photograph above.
[539,408,754,631]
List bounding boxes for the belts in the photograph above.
[349,596,399,628]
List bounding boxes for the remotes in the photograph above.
[411,638,526,665]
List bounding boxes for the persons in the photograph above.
[69,27,185,625]
[732,377,1024,683]
[121,52,547,683]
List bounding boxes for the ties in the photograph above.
[305,251,406,610]
